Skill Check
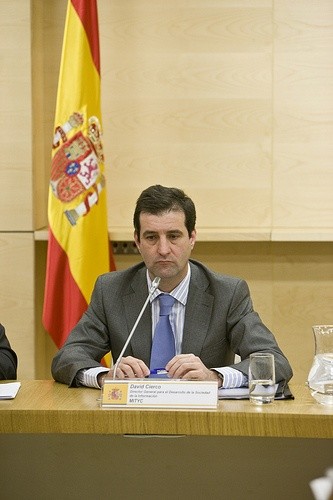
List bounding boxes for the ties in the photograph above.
[149,294,175,378]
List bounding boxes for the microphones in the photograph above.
[113,276,161,379]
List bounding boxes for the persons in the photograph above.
[50,183,294,390]
[0,323,19,381]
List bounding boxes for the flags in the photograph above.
[40,0,119,370]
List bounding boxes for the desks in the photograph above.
[0,380,333,500]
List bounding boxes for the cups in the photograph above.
[248,353,276,405]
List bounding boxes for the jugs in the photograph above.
[307,325,333,405]
